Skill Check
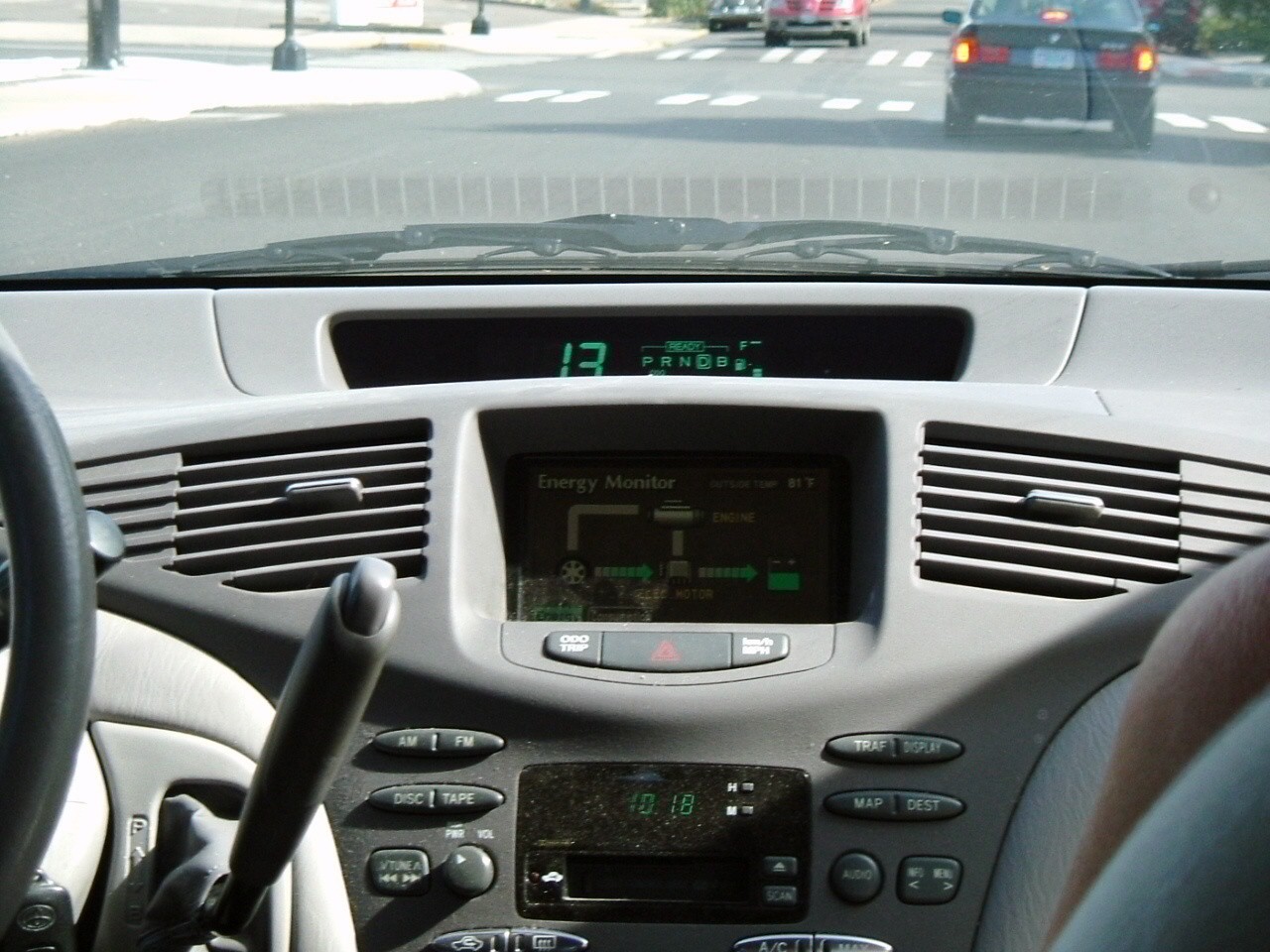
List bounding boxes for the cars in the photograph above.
[1140,1,1204,56]
[707,0,764,34]
[761,0,873,50]
[942,0,1160,148]
